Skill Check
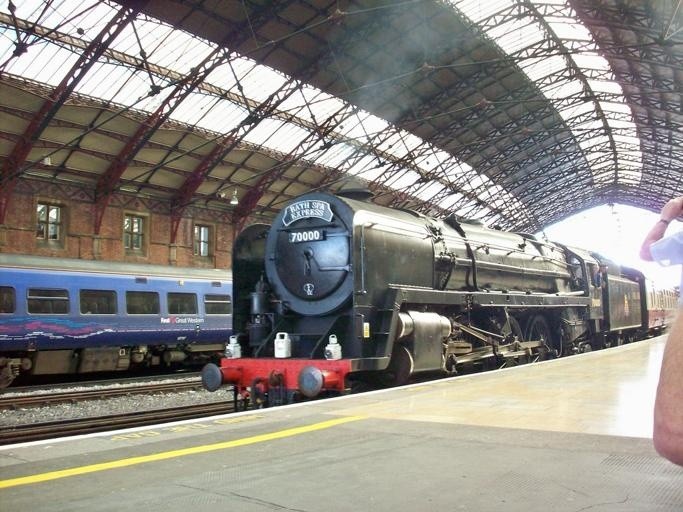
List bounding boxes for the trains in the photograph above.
[193,188,679,413]
[0,263,234,389]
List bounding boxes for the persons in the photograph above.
[638,195,683,267]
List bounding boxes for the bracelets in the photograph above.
[657,218,669,225]
